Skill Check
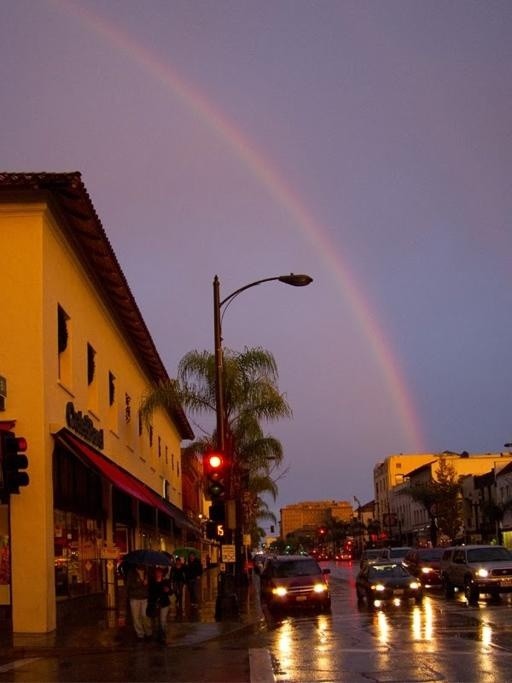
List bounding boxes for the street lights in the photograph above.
[212,269,313,624]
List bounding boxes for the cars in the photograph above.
[355,559,423,607]
[299,547,353,562]
[252,551,264,570]
[360,548,384,567]
[54,558,82,585]
[405,547,446,585]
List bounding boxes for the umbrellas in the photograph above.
[122,548,168,568]
[173,546,202,563]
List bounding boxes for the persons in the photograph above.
[118,551,204,642]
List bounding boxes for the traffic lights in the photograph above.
[207,519,225,539]
[204,449,229,499]
[0,429,30,494]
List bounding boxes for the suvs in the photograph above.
[378,545,413,574]
[440,542,512,602]
[260,551,332,615]
